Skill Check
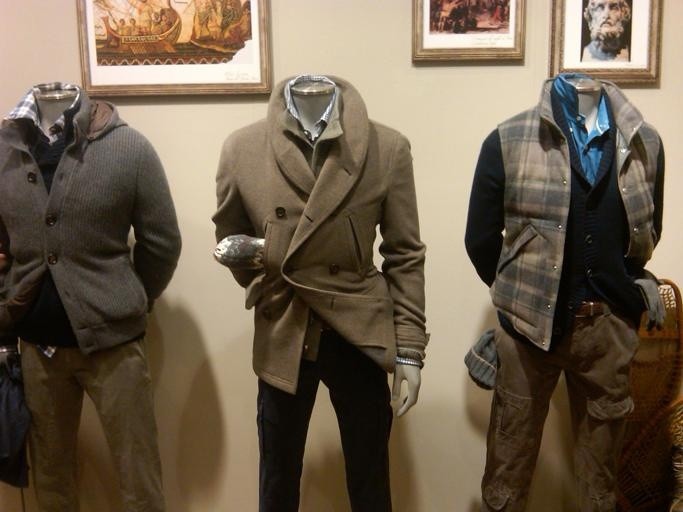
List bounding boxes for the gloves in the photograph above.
[634,279,667,332]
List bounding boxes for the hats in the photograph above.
[465,330,497,390]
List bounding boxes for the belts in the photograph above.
[574,302,603,319]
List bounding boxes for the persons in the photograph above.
[212,74,430,512]
[431,0,509,33]
[116,9,171,36]
[0,82,183,512]
[194,0,252,40]
[580,0,632,63]
[465,75,664,512]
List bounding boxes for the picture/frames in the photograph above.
[75,0,272,98]
[548,0,663,89]
[411,0,527,63]
